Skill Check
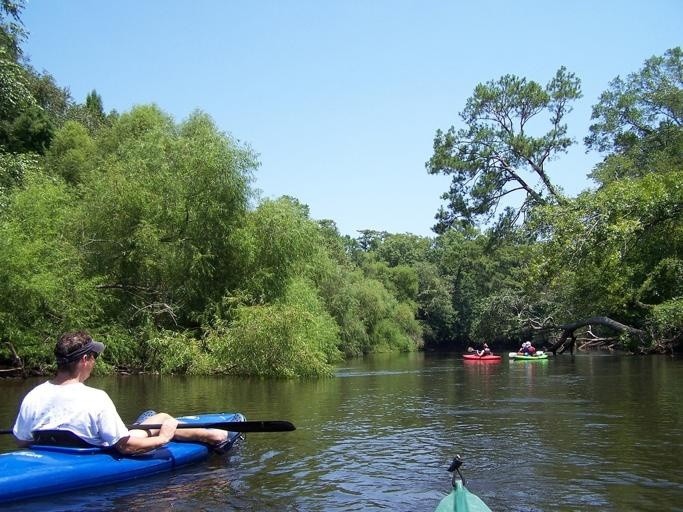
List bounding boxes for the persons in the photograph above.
[518,340,536,357]
[517,343,527,353]
[477,342,493,358]
[13,330,244,457]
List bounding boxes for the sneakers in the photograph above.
[211,431,242,456]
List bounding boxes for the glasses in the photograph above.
[56,336,104,366]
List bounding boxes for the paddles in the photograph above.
[467,347,483,353]
[0,420,296,433]
[507,352,523,357]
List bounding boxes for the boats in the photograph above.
[461,352,502,360]
[0,409,248,504]
[508,350,549,360]
[434,453,496,511]
